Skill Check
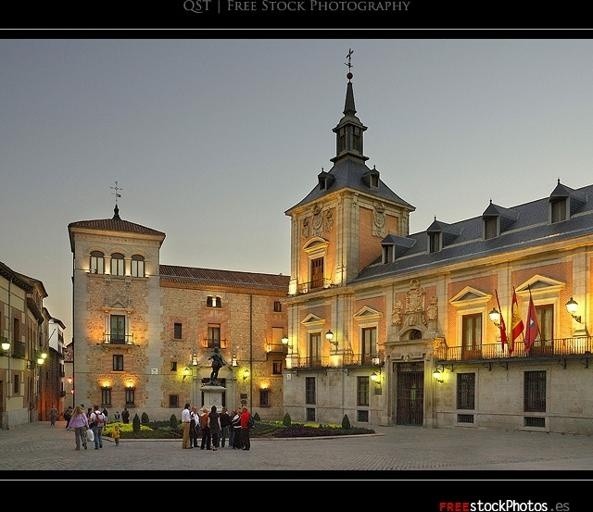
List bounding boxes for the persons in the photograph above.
[181,402,250,450]
[121,407,129,423]
[47,405,57,427]
[207,346,226,385]
[111,425,120,445]
[63,403,107,449]
[114,411,120,420]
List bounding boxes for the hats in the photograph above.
[69,407,72,409]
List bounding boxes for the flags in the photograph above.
[524,292,538,355]
[507,291,524,352]
[495,290,507,354]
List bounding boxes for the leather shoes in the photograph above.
[182,445,250,451]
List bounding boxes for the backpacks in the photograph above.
[94,412,104,428]
[248,415,254,427]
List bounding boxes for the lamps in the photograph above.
[280,334,293,350]
[243,368,250,379]
[370,351,385,368]
[324,328,338,349]
[486,306,501,329]
[430,363,448,384]
[564,295,581,324]
[0,335,12,356]
[181,366,190,380]
[369,369,381,387]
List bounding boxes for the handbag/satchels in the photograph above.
[218,432,223,438]
[195,424,202,438]
[204,427,210,436]
